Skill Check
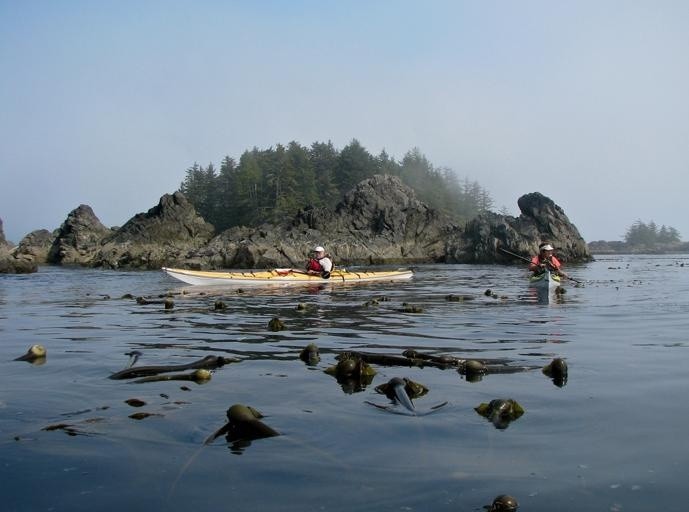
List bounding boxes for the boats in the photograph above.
[161,267,413,286]
[528,271,561,287]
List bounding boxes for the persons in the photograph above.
[527,243,568,279]
[306,246,333,279]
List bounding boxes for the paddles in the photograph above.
[292,271,330,279]
[501,248,581,283]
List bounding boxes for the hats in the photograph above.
[311,246,325,253]
[540,244,553,252]
